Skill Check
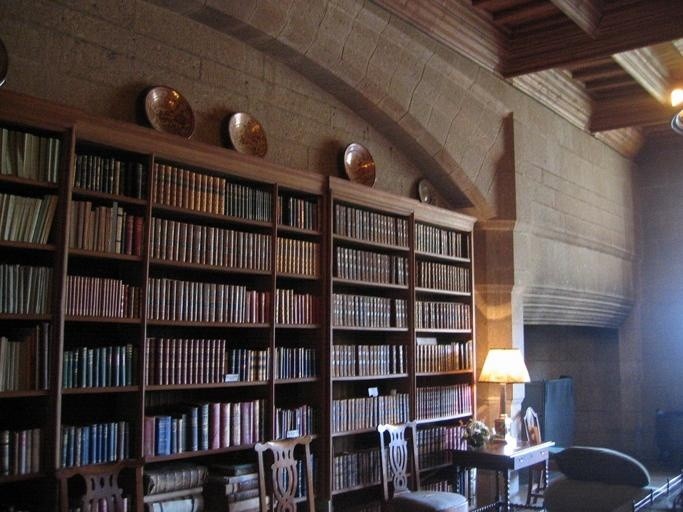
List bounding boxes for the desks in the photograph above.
[444,441,556,511]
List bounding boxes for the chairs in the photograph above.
[376,418,469,511]
[253,433,325,511]
[523,405,565,505]
[55,458,147,511]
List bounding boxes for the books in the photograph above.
[333,204,409,247]
[0,264,54,313]
[282,454,317,497]
[0,194,58,244]
[276,289,320,324]
[275,347,316,379]
[148,278,270,323]
[89,495,131,512]
[415,260,470,292]
[74,154,146,199]
[146,338,271,386]
[63,344,137,388]
[143,462,270,512]
[417,424,470,469]
[417,301,471,329]
[417,384,472,420]
[145,400,265,456]
[333,345,409,377]
[335,445,410,490]
[153,163,272,222]
[65,276,143,318]
[0,324,50,391]
[276,238,322,276]
[276,404,319,439]
[0,128,64,182]
[61,421,131,467]
[416,337,473,372]
[278,196,317,231]
[333,498,383,512]
[334,246,410,285]
[70,200,145,255]
[0,428,46,476]
[333,393,409,433]
[415,223,469,258]
[333,293,408,328]
[151,218,273,271]
[422,467,474,506]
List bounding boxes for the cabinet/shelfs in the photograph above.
[0,87,154,484]
[330,178,474,512]
[144,129,329,512]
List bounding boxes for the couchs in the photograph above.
[543,441,663,511]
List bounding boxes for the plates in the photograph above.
[341,142,375,186]
[229,112,266,160]
[0,38,10,91]
[416,178,434,203]
[143,86,195,142]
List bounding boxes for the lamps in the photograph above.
[481,347,532,446]
